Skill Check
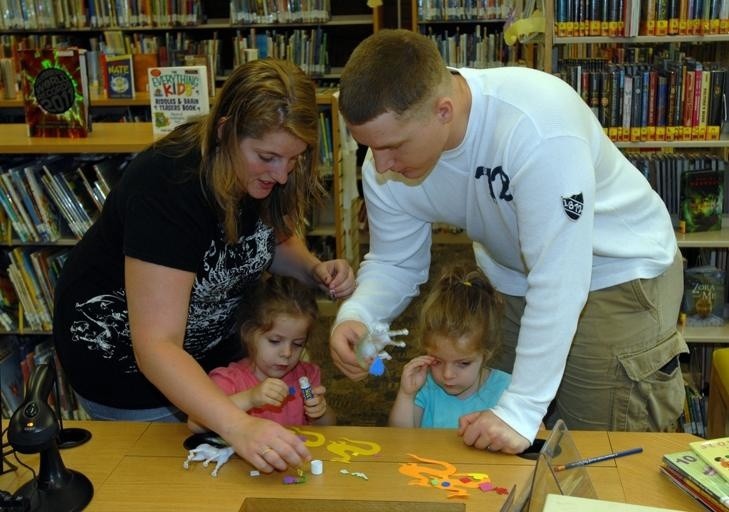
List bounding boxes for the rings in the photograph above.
[260,448,272,457]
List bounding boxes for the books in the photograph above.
[0,33,104,138]
[656,437,729,512]
[553,0,729,38]
[680,246,728,269]
[0,154,111,246]
[556,42,729,142]
[0,245,67,333]
[622,149,727,234]
[229,0,332,26]
[681,341,721,437]
[0,1,203,27]
[420,24,545,72]
[318,107,334,170]
[0,336,95,421]
[416,0,544,21]
[231,27,332,75]
[84,30,221,99]
[684,266,726,328]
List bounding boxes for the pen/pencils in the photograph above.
[554,446,644,473]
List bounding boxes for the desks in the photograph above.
[0,411,718,512]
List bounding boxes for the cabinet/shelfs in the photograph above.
[0,0,358,310]
[368,1,729,285]
[0,121,305,424]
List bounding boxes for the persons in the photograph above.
[325,28,691,456]
[53,54,358,475]
[386,269,512,429]
[187,270,336,435]
[691,286,724,326]
